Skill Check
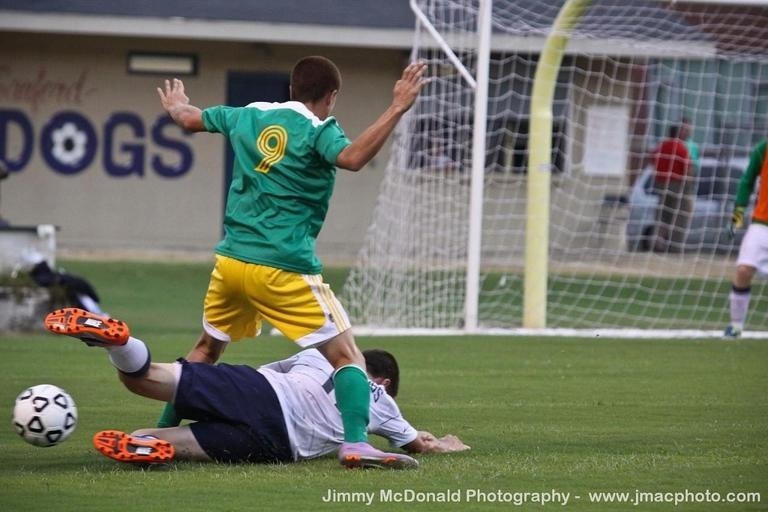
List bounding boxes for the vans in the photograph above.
[625,155,760,254]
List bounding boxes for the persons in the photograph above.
[678,118,701,173]
[156,54,432,471]
[43,305,472,465]
[720,139,768,338]
[646,119,698,255]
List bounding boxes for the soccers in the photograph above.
[12,384,79,447]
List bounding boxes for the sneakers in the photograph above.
[720,322,743,344]
[91,430,177,466]
[337,441,420,472]
[42,307,131,347]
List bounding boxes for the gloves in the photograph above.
[724,205,747,240]
